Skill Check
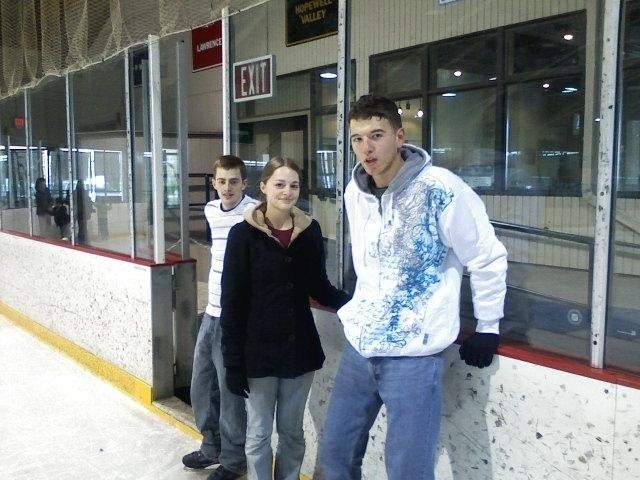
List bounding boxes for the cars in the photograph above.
[59,176,118,194]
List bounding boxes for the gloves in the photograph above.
[226,368,250,398]
[460,334,498,369]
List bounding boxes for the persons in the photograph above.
[216,154,353,479]
[93,185,114,241]
[68,180,91,245]
[34,177,52,235]
[178,154,265,479]
[50,198,71,242]
[311,89,510,479]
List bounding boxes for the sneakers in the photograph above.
[207,466,239,480]
[183,451,218,468]
